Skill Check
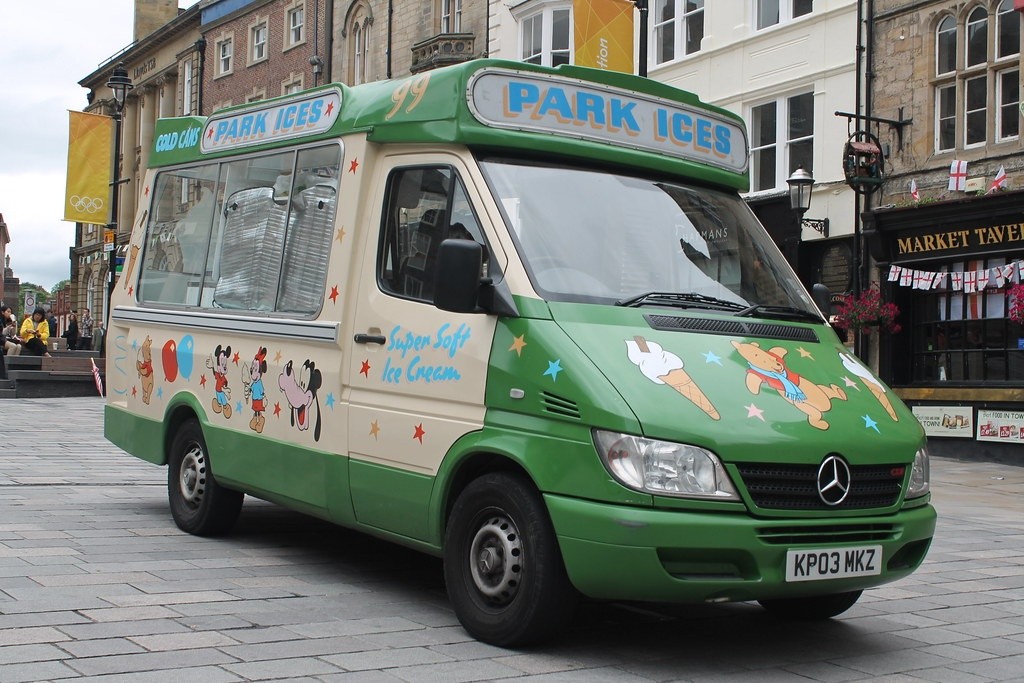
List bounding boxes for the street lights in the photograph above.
[104,59,132,333]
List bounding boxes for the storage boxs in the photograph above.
[47,337,67,352]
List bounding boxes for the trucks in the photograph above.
[98,57,940,648]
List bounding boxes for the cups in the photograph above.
[53,343,57,350]
[942,414,950,428]
[10,314,15,321]
[948,418,957,429]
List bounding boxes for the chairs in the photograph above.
[397,208,446,302]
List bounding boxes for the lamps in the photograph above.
[787,165,830,237]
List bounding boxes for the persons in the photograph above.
[47,314,57,337]
[76,309,94,350]
[62,313,78,350]
[20,307,52,357]
[0,304,21,355]
[92,322,106,350]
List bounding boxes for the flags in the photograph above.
[888,265,901,281]
[912,271,947,289]
[911,179,920,201]
[948,160,967,190]
[951,272,963,290]
[993,267,1005,288]
[900,268,912,286]
[1003,263,1015,282]
[978,270,990,291]
[80,245,129,264]
[1019,261,1024,280]
[986,166,1007,194]
[964,271,975,293]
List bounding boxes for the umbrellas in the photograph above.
[91,357,103,398]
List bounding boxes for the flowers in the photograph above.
[832,286,901,336]
